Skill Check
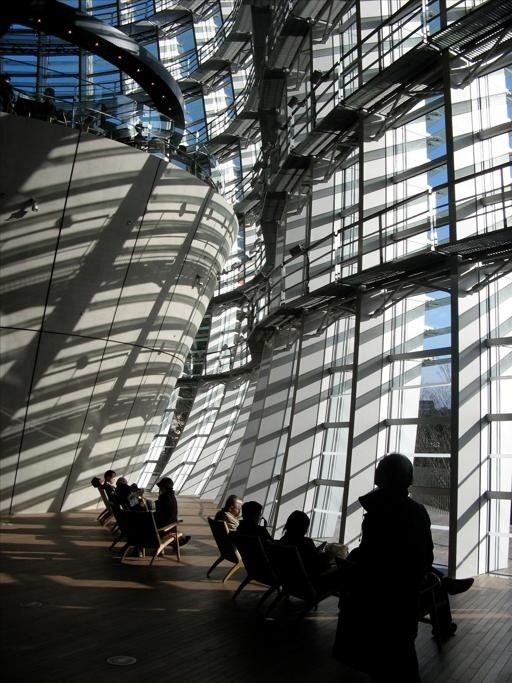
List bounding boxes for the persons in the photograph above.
[91,477,103,489]
[146,477,178,536]
[103,470,117,496]
[335,454,435,683]
[114,477,132,504]
[280,510,348,593]
[347,511,474,636]
[238,500,327,573]
[128,484,190,548]
[214,495,244,535]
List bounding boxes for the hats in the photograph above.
[374,455,414,486]
[156,477,173,492]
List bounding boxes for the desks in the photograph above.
[15,98,56,122]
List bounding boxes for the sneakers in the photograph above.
[432,622,457,636]
[445,576,474,595]
